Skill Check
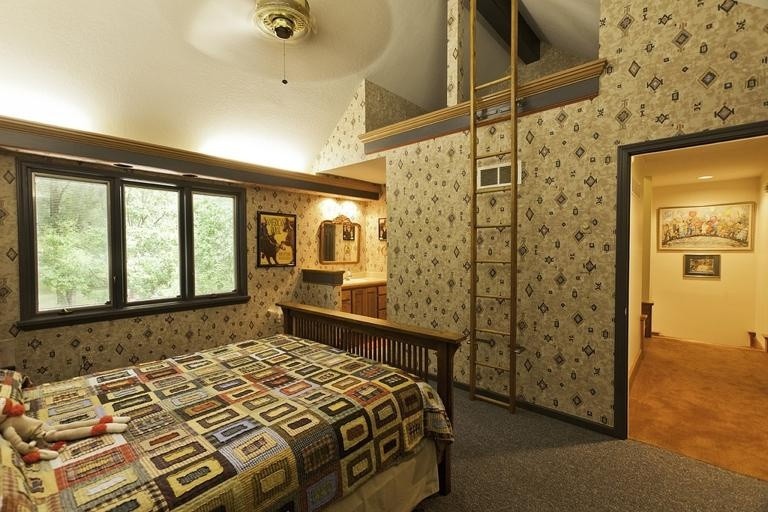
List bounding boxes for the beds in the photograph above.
[0,301,463,512]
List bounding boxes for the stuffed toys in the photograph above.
[1,393,131,465]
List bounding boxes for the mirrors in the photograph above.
[320,214,361,264]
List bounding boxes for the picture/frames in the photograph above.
[257,209,296,268]
[378,217,386,240]
[655,201,755,277]
[342,221,355,241]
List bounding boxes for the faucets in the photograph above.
[344,272,352,279]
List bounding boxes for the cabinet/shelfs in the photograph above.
[340,285,386,321]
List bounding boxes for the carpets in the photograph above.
[417,378,766,511]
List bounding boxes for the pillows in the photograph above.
[0,363,41,512]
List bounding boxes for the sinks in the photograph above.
[343,279,367,284]
